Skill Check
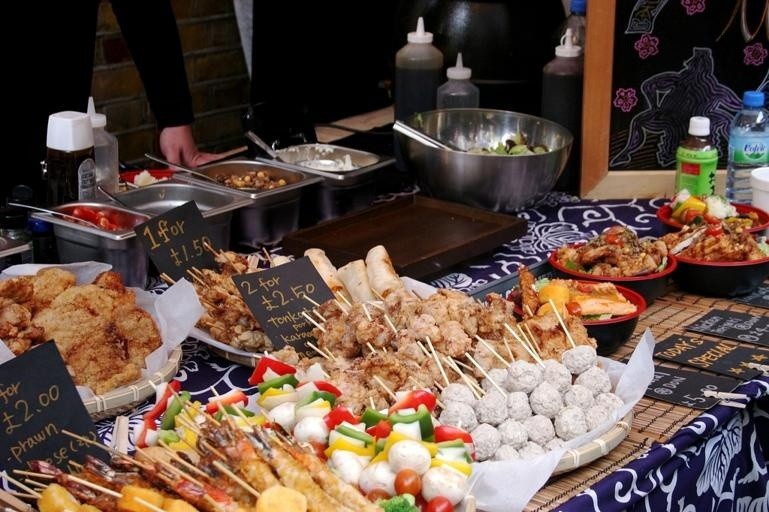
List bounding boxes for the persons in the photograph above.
[1,1,224,207]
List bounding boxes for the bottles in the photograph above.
[541,0,587,180]
[436,54,481,110]
[87,95,119,204]
[674,115,720,201]
[45,110,97,207]
[394,17,445,173]
[724,90,769,206]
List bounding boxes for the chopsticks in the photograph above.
[392,120,454,152]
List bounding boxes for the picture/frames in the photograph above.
[580,0,769,200]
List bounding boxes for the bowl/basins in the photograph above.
[548,242,678,306]
[394,108,574,214]
[507,277,648,356]
[671,233,768,299]
[656,203,769,243]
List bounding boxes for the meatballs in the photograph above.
[267,345,625,508]
